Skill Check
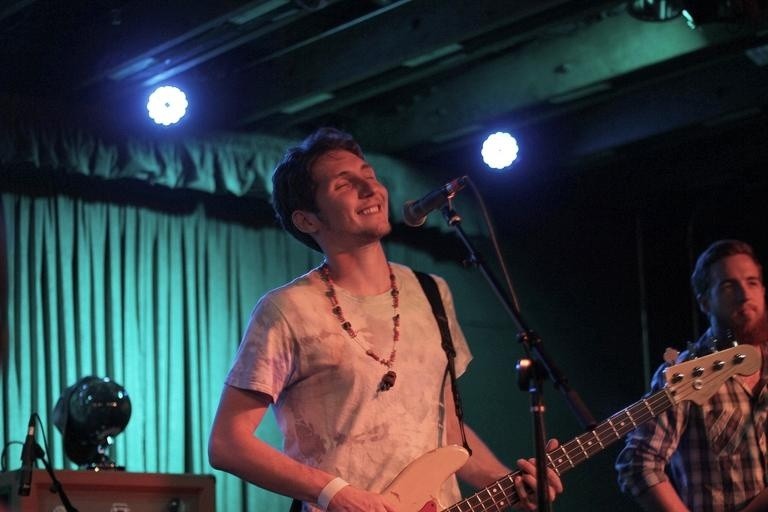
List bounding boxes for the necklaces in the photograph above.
[320,261,401,392]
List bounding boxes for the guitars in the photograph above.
[372,331,762,511]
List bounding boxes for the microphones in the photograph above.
[402,176,465,227]
[17,423,39,495]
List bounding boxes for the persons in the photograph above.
[207,128,562,510]
[615,238,768,512]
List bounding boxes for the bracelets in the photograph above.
[317,477,349,509]
